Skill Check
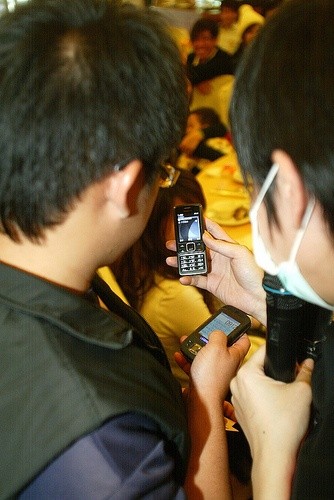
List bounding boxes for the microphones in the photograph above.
[261,272,302,383]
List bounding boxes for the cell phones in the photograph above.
[179,304,252,363]
[173,203,208,276]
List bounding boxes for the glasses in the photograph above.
[114,158,181,187]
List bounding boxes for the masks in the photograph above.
[249,159,334,311]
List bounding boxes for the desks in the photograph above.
[195,150,267,332]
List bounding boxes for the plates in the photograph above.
[207,201,250,226]
[234,171,252,184]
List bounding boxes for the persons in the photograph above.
[166,0,334,500]
[0,0,334,500]
